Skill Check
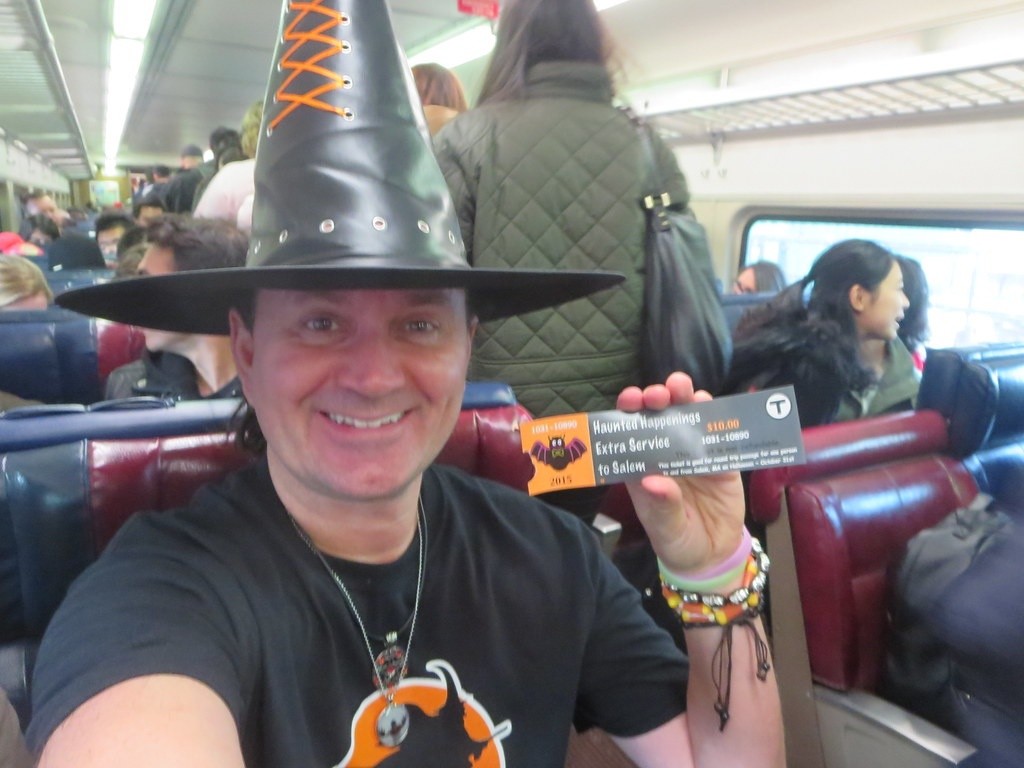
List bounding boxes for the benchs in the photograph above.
[0,219,533,721]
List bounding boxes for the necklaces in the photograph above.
[291,510,425,745]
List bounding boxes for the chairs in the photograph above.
[916,344,1024,507]
[750,411,982,768]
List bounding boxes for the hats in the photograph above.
[54,0,626,334]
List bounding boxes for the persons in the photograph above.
[410,62,466,136]
[159,146,205,214]
[726,239,909,523]
[735,262,784,295]
[0,254,51,314]
[19,187,784,768]
[835,256,931,425]
[146,165,170,204]
[194,102,264,228]
[133,166,155,203]
[94,214,135,267]
[20,217,58,246]
[210,126,236,153]
[136,215,245,399]
[20,192,62,229]
[114,228,148,276]
[190,137,242,214]
[432,1,692,419]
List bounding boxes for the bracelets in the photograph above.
[659,530,769,731]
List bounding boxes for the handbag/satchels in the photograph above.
[623,104,733,400]
